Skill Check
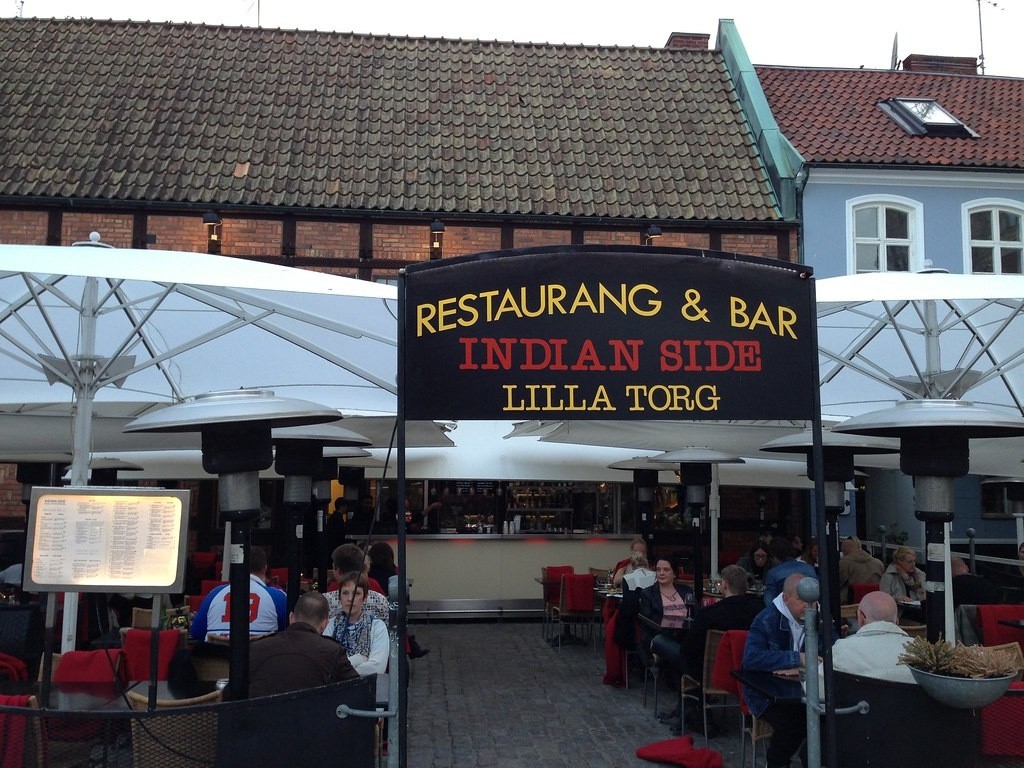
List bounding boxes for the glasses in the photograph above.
[753,554,768,560]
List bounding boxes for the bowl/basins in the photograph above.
[597,583,612,591]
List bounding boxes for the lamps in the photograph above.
[644,224,663,239]
[429,217,446,235]
[202,209,220,226]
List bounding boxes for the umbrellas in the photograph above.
[0,232,458,656]
[503,259,1024,648]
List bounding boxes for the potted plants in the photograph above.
[895,630,1019,711]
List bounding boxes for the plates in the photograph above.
[608,591,624,594]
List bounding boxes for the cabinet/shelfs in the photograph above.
[505,485,574,534]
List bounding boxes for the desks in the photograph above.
[98,681,218,768]
[535,577,589,647]
[728,669,806,703]
[0,678,139,709]
[638,613,694,632]
[592,590,623,599]
[898,601,923,612]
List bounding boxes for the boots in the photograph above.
[409,635,430,659]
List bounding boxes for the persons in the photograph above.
[0,497,441,768]
[604,531,1024,768]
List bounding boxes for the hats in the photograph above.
[248,546,267,568]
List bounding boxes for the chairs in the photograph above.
[0,552,1024,768]
